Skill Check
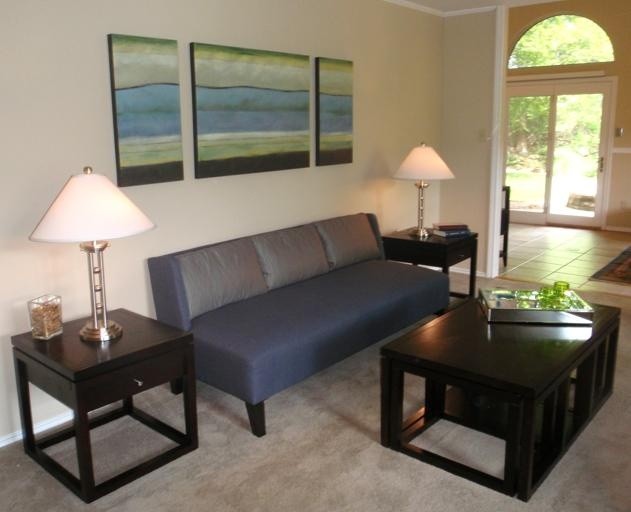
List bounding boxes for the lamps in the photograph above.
[391,143,456,238]
[28,165,156,343]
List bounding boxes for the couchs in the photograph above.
[147,212,450,438]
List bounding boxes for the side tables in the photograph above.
[383,226,478,318]
[11,308,199,504]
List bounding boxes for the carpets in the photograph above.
[588,245,631,286]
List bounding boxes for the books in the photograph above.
[432,229,471,238]
[433,223,469,231]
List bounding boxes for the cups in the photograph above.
[534,281,571,310]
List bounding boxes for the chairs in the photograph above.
[500,186,509,267]
